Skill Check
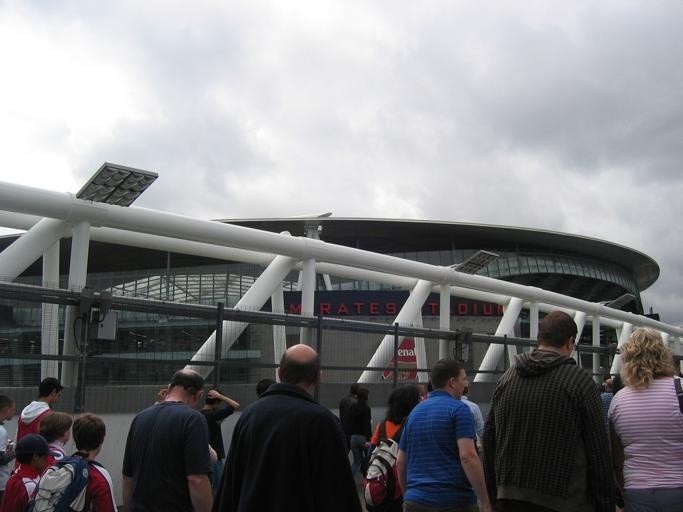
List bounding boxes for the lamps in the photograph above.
[603,293,636,309]
[76,163,158,207]
[451,250,500,276]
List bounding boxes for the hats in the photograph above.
[14,434,58,457]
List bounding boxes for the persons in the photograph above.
[344,386,373,478]
[370,310,683,511]
[0,377,118,511]
[121,344,363,511]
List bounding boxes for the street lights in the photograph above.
[434,248,502,362]
[592,292,638,380]
[58,159,159,386]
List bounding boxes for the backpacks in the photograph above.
[362,419,407,510]
[27,452,104,511]
